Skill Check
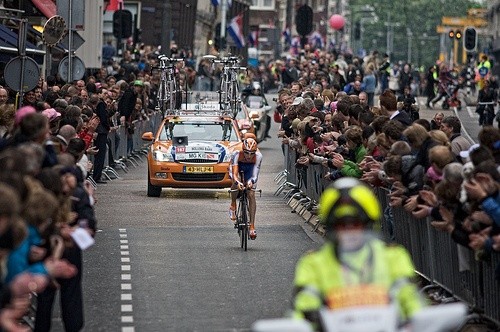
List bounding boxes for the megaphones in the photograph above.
[173,136,189,146]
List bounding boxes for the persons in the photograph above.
[0,44,500,332]
[228,137,263,238]
[291,175,432,332]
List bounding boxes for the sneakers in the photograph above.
[228,207,237,220]
[249,227,257,240]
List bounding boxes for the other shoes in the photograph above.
[98,181,107,183]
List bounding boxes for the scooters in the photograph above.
[249,300,469,332]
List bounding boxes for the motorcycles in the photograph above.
[244,95,273,144]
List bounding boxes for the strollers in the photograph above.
[436,79,469,111]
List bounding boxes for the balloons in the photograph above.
[329,14,346,31]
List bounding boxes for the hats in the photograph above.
[42,108,61,122]
[303,116,318,123]
[459,143,480,157]
[289,118,300,128]
[134,80,143,87]
[292,97,303,105]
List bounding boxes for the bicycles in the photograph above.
[476,99,497,128]
[209,51,250,120]
[148,55,188,121]
[227,183,262,252]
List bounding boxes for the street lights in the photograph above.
[383,21,401,52]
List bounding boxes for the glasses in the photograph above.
[244,151,255,154]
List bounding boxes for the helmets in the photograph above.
[316,175,380,224]
[242,138,257,151]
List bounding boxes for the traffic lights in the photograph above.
[132,27,143,43]
[463,26,478,53]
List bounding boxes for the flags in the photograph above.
[32,0,56,17]
[103,0,124,11]
[226,13,247,47]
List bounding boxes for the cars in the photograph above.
[181,101,260,136]
[141,103,257,197]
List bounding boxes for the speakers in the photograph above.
[295,5,313,36]
[113,9,132,38]
